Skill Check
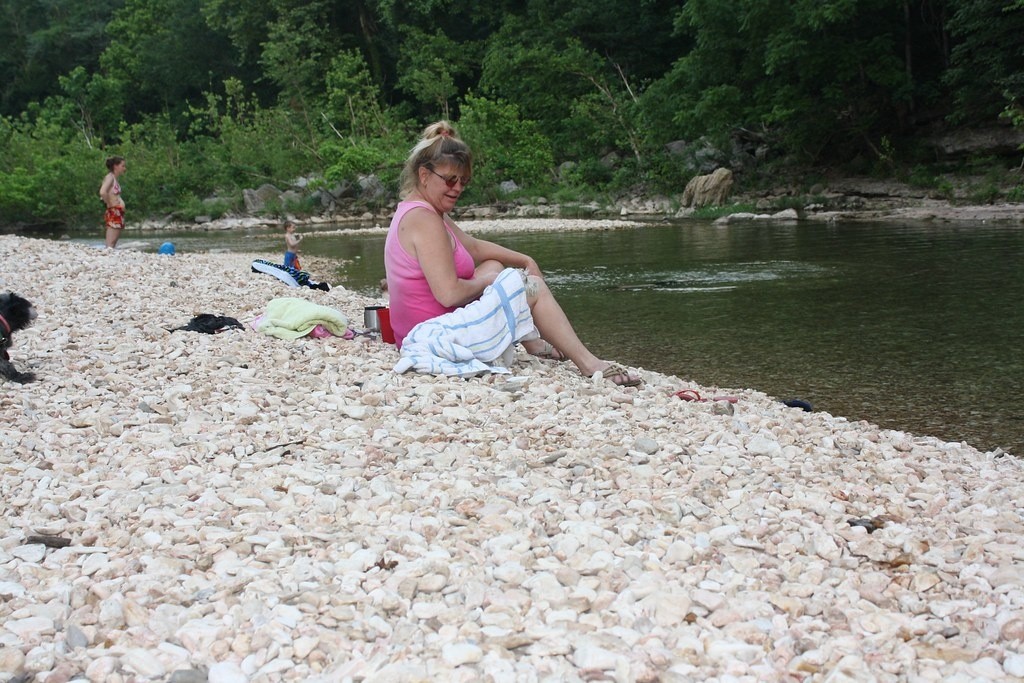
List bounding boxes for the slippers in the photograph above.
[583,362,641,388]
[527,343,570,362]
[668,388,738,403]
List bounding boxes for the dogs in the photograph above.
[0,292,38,383]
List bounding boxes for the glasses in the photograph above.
[428,168,471,185]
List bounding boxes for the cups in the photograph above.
[378,307,396,344]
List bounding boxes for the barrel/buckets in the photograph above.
[365,306,386,331]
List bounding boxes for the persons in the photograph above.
[284,221,302,269]
[385,120,641,387]
[99,156,126,249]
